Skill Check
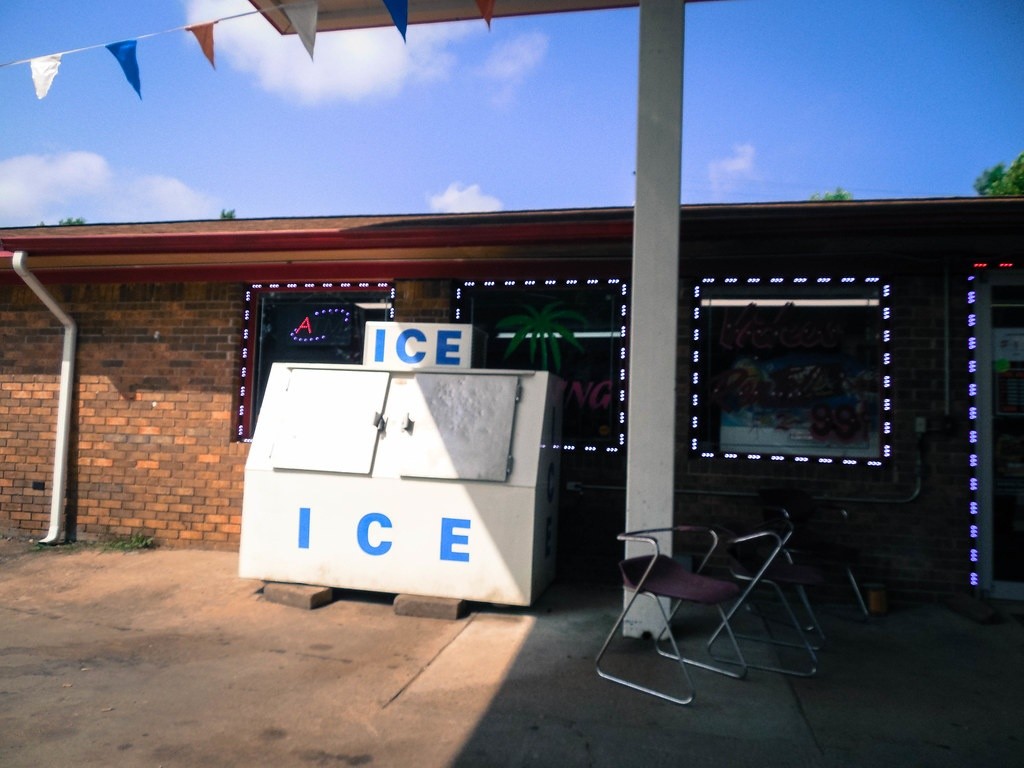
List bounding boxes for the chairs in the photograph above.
[757,488,871,632]
[594,524,784,707]
[702,515,828,679]
